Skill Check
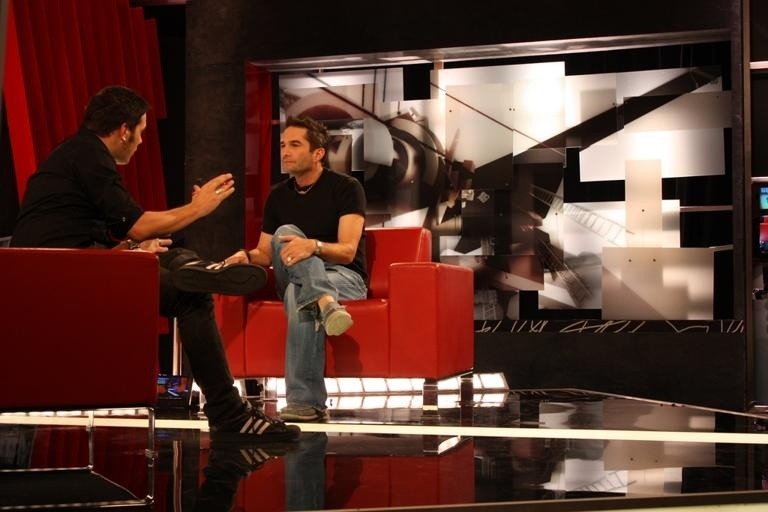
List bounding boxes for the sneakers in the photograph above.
[277,401,327,420]
[205,406,303,444]
[293,433,329,456]
[172,260,266,296]
[320,302,355,338]
[208,445,294,480]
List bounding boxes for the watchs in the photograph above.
[315,239,324,256]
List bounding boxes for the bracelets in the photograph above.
[240,248,252,264]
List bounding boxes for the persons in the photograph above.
[7,85,303,445]
[147,432,289,510]
[197,113,371,426]
[280,428,329,511]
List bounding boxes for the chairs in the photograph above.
[1,233,164,510]
[195,223,478,416]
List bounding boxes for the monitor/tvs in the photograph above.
[151,372,195,409]
[271,37,733,322]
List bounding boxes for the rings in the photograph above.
[215,189,222,194]
[287,255,292,262]
